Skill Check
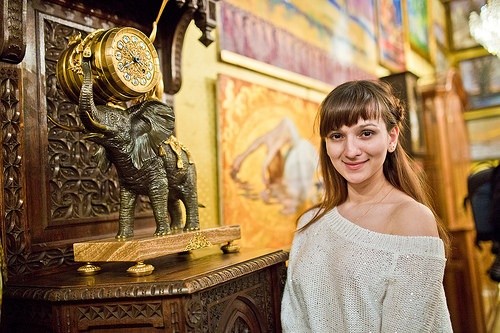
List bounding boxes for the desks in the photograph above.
[14,248,290,333]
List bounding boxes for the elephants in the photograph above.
[46,61,206,239]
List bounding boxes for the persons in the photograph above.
[280,80,454,333]
